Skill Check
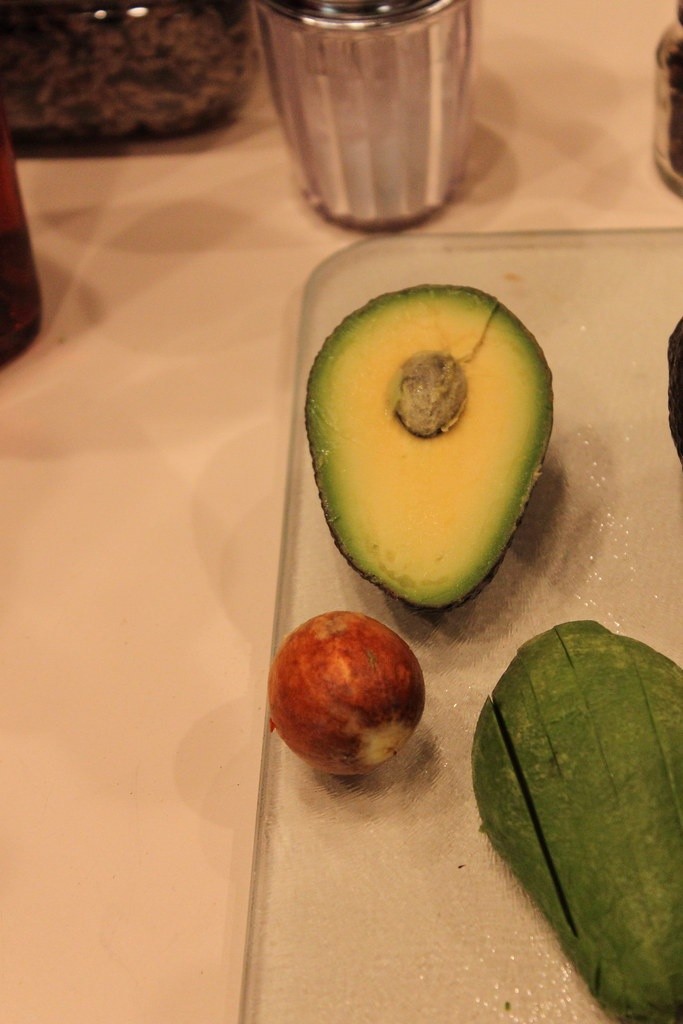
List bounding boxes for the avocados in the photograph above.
[470,619,683,1024]
[304,283,554,614]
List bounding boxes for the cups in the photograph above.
[0,111,45,368]
[237,222,683,1024]
[247,0,475,232]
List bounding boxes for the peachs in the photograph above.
[268,611,426,775]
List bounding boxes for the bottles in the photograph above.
[650,0,683,200]
